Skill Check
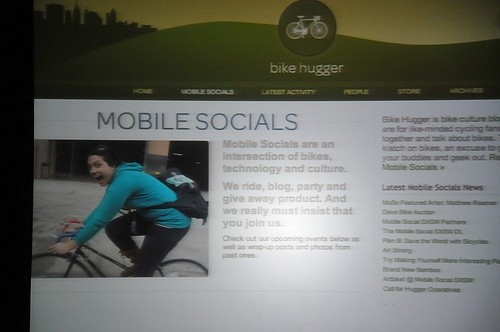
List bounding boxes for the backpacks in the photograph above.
[121,168,208,219]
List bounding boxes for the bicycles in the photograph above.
[33,220,208,277]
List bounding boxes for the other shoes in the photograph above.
[120,256,138,276]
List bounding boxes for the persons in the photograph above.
[50,145,192,277]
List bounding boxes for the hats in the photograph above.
[89,144,116,167]
